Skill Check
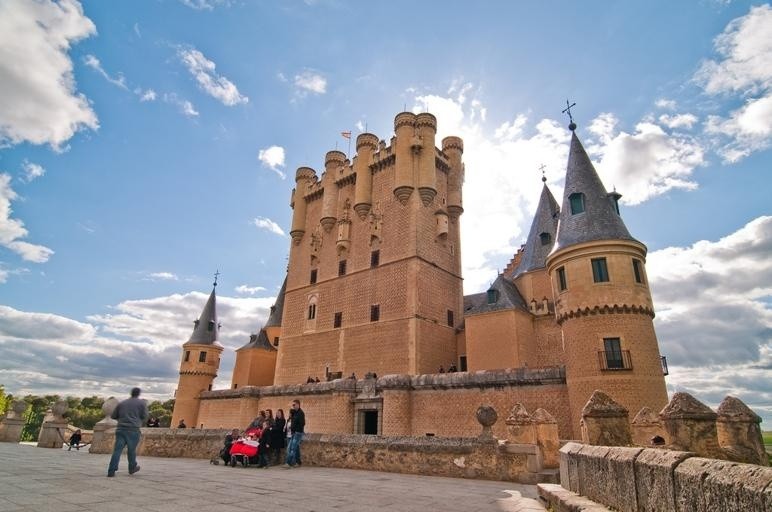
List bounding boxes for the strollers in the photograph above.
[229,426,264,468]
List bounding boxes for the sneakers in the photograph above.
[253,462,301,469]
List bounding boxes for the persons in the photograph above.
[68,429,82,451]
[107,386,148,477]
[178,417,187,427]
[220,399,306,470]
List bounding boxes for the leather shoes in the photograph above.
[129,465,141,474]
[108,470,115,477]
